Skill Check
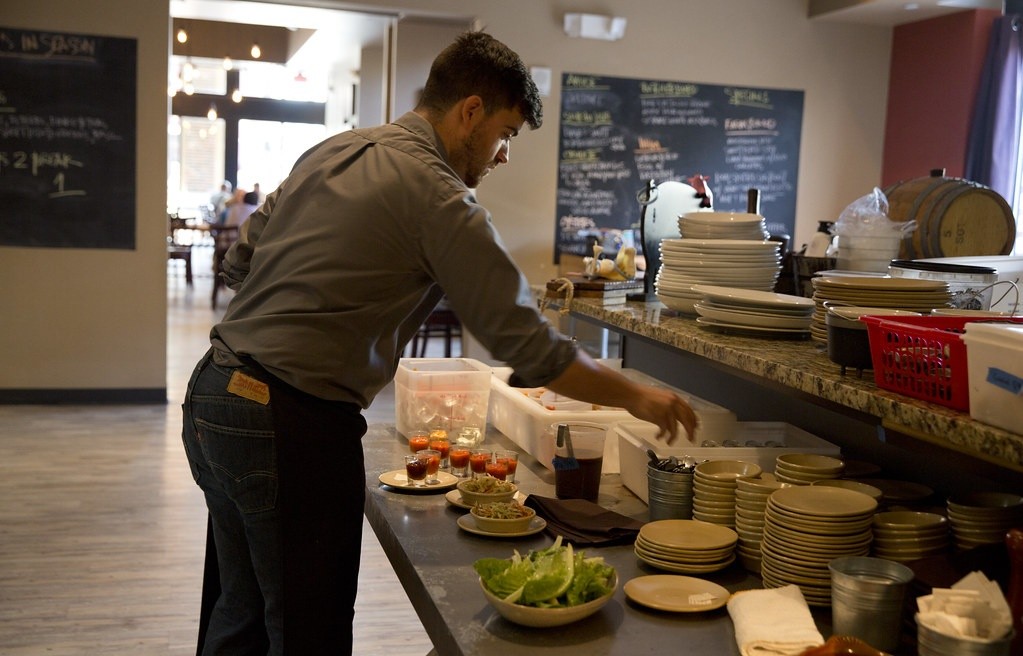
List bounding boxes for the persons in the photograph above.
[181,31,697,656]
[211,181,264,236]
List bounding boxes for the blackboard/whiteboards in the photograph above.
[553,74,804,268]
[1,24,140,250]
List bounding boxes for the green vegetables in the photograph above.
[473,534,616,608]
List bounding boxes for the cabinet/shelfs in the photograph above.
[361,285,1023,656]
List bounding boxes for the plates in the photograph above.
[690,284,815,334]
[447,489,547,537]
[654,213,784,310]
[622,454,1022,612]
[379,468,458,488]
[811,277,957,342]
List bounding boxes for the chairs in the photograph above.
[209,225,239,311]
[169,215,194,282]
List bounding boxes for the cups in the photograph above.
[550,420,607,506]
[830,554,914,652]
[914,614,1015,656]
[400,392,518,483]
[647,456,694,521]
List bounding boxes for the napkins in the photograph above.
[523,494,646,548]
[727,585,825,656]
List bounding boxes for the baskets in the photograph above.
[857,315,1022,414]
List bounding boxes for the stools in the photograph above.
[401,295,463,358]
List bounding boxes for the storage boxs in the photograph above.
[792,230,1023,435]
[395,358,842,507]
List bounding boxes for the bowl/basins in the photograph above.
[458,477,536,533]
[479,557,618,626]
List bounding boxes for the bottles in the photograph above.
[804,219,836,256]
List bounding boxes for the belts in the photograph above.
[240,354,361,414]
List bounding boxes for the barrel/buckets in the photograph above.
[888,256,1023,312]
[794,223,902,297]
[877,175,1015,258]
[823,281,1023,377]
